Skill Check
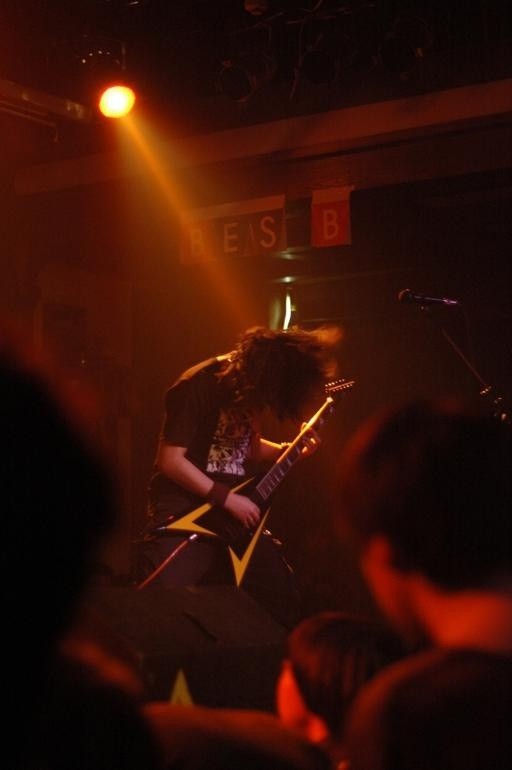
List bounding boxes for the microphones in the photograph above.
[395,287,460,310]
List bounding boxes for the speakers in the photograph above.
[96,579,289,654]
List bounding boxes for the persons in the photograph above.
[149,327,318,630]
[273,605,397,745]
[341,400,512,770]
[1,350,166,770]
[63,624,330,769]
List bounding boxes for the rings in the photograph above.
[249,518,254,523]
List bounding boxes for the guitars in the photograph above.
[149,377,354,593]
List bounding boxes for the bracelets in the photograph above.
[205,480,231,507]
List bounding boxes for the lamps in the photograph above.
[70,24,137,122]
